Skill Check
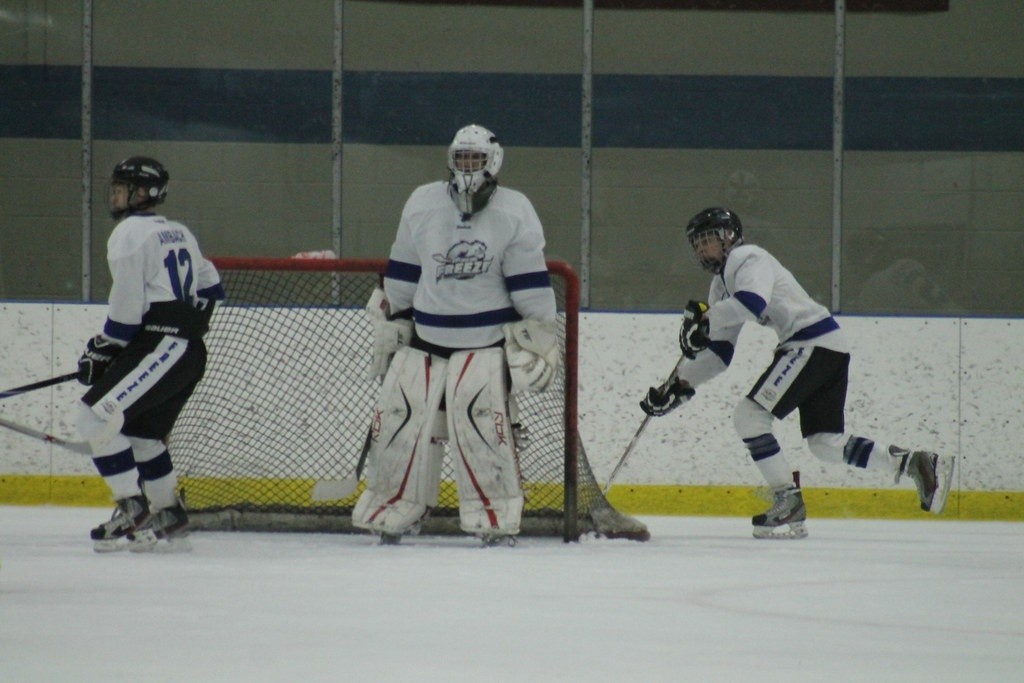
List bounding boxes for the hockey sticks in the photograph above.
[1,370,80,399]
[601,351,686,498]
[312,424,372,501]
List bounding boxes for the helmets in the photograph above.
[447,124,504,192]
[685,207,743,271]
[108,156,170,221]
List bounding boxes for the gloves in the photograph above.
[76,336,122,387]
[679,299,710,361]
[640,378,696,418]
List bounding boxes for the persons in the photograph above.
[350,126,557,551]
[639,206,956,540]
[77,155,226,552]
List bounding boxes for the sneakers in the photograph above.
[751,475,808,541]
[90,499,150,553]
[907,450,956,515]
[126,495,192,554]
